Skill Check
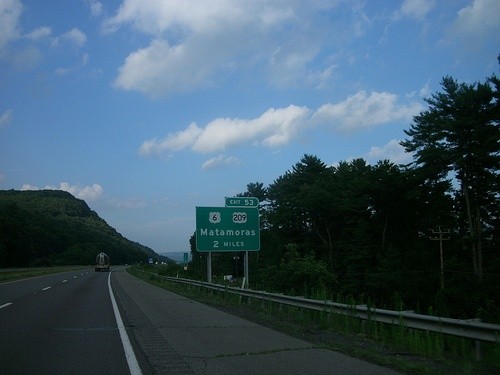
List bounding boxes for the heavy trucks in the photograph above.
[95,253,110,272]
